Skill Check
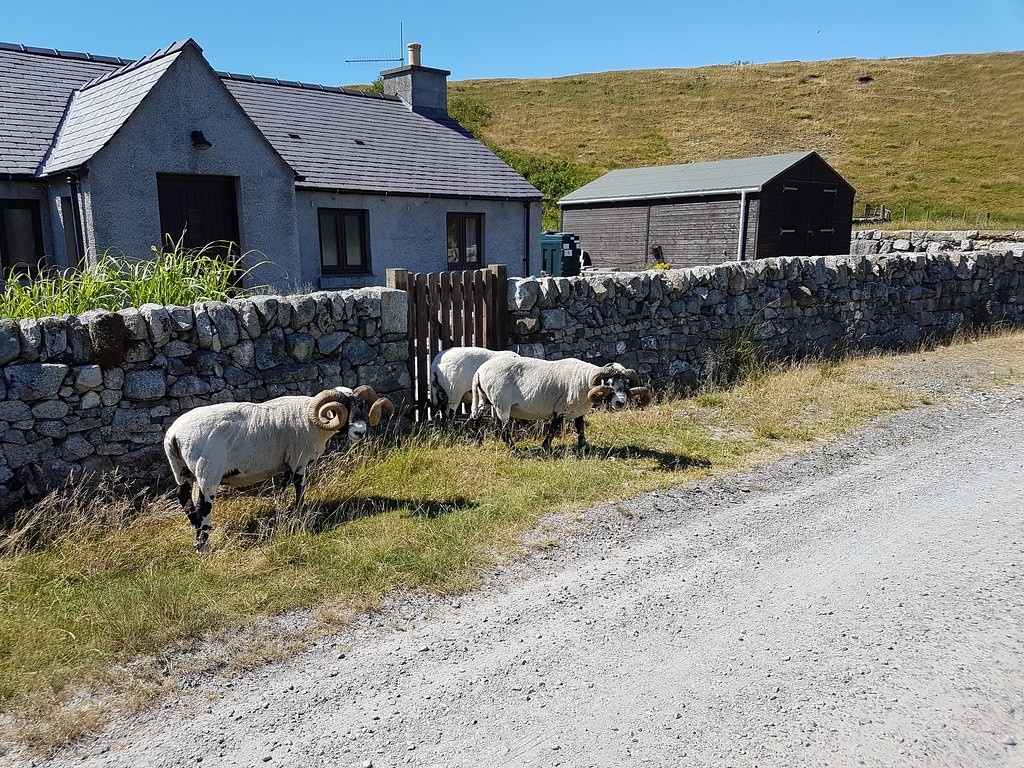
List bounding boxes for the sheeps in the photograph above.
[163,384,395,554]
[428,347,648,459]
